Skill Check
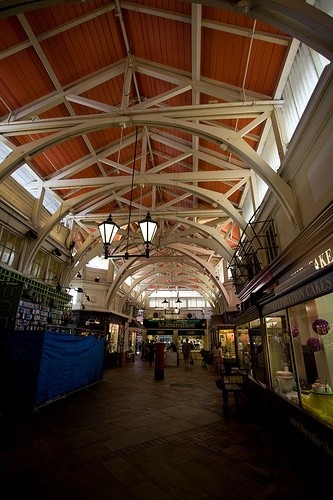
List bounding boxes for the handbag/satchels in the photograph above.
[190,356,194,365]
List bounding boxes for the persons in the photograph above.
[141,338,223,375]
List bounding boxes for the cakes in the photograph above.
[312,383,332,394]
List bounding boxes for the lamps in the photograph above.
[33,272,91,310]
[175,292,182,304]
[28,229,38,241]
[97,125,159,259]
[163,298,169,303]
[52,247,61,256]
